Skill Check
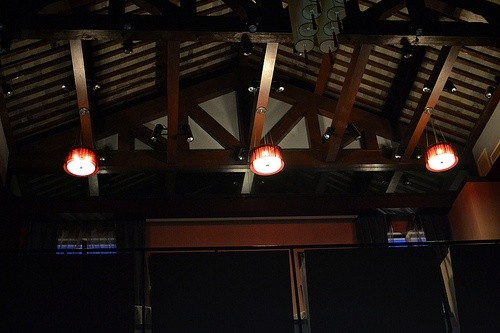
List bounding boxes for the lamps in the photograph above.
[0,0,500,188]
